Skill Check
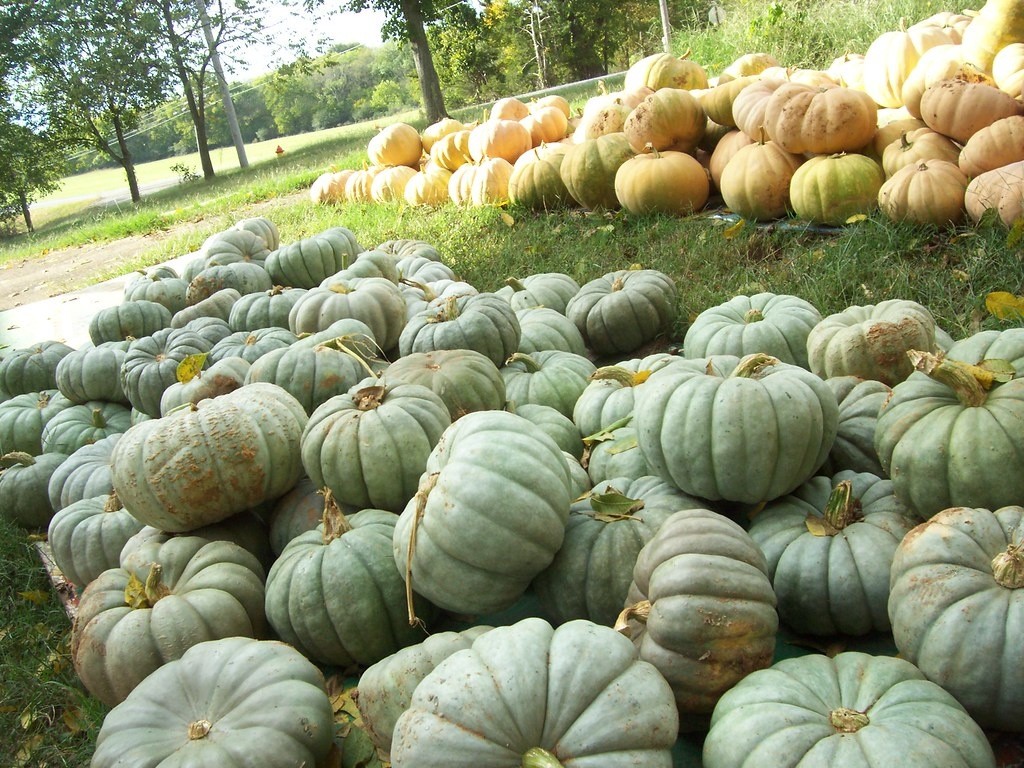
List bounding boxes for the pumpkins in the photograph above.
[0,216,1024,768]
[309,0,1024,231]
